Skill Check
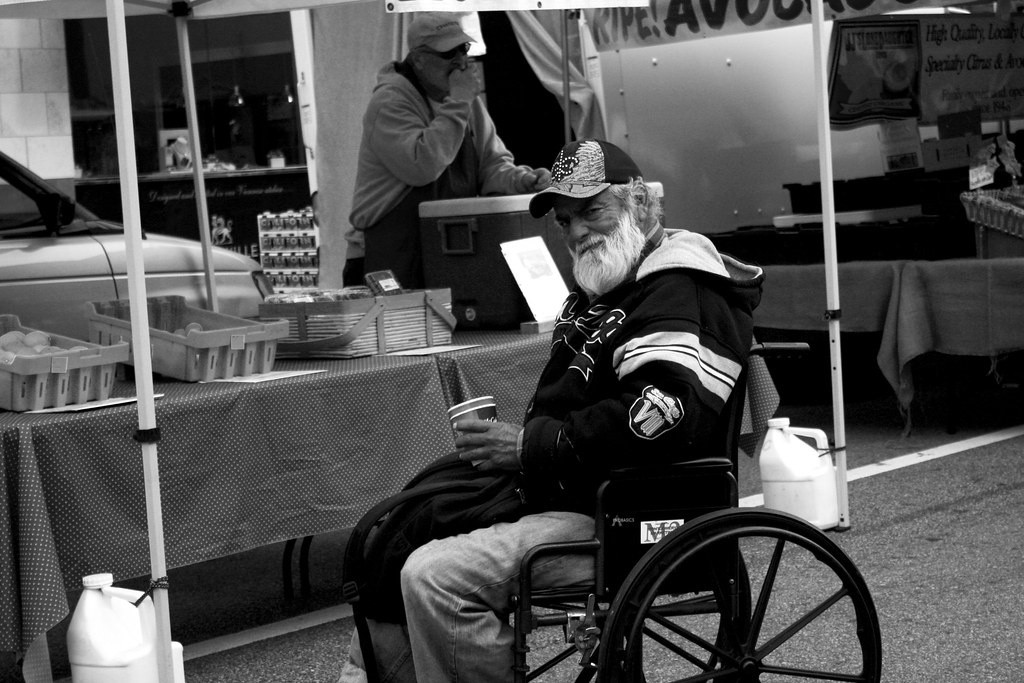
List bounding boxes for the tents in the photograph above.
[0,1,852,683]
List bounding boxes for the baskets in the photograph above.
[258,286,456,358]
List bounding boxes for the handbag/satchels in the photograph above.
[343,449,574,626]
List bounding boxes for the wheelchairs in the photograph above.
[339,341,885,683]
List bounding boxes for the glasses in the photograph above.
[419,42,471,60]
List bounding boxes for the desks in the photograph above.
[300,330,555,585]
[750,260,1024,432]
[0,354,458,683]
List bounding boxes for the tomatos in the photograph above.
[0,330,90,356]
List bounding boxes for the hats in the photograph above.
[407,11,477,53]
[529,136,644,218]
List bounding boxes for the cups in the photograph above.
[447,395,498,468]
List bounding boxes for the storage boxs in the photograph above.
[420,180,668,331]
[78,294,290,382]
[704,119,1024,265]
[1,315,130,412]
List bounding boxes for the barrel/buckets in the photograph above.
[64,574,158,683]
[756,416,840,530]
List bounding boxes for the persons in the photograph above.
[341,139,766,683]
[342,14,553,288]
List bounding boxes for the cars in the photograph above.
[0,151,276,343]
